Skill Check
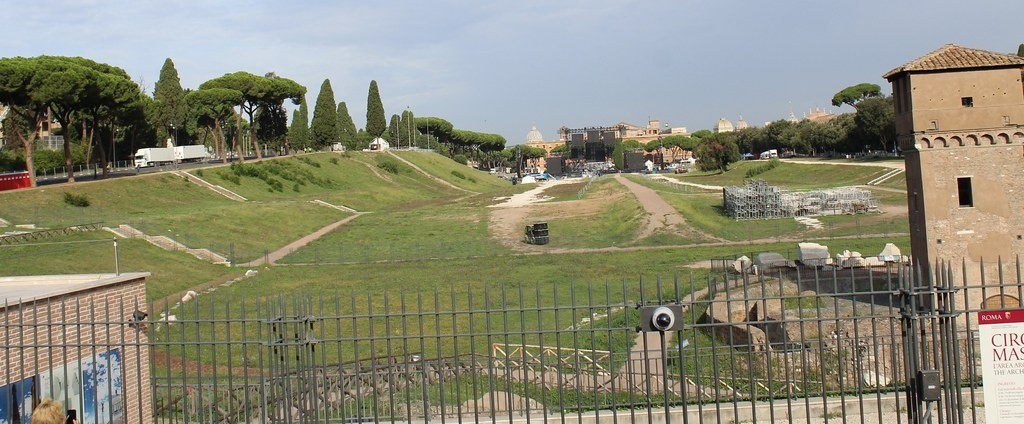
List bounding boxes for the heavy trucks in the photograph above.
[134,147,175,168]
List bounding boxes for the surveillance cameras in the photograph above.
[133,310,148,320]
[652,307,675,330]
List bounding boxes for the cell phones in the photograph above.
[67,409,76,424]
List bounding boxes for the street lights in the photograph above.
[169,123,178,146]
[113,127,119,169]
[225,120,233,162]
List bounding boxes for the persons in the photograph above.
[29,398,79,424]
